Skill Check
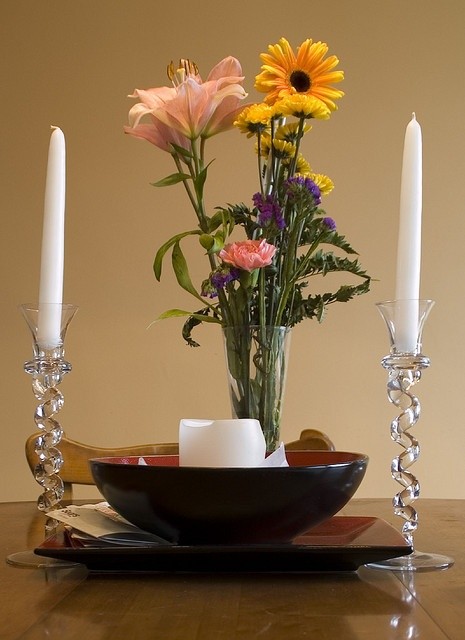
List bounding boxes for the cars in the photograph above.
[178,416,267,467]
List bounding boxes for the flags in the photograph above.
[219,324,292,459]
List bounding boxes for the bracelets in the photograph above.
[89,450,370,545]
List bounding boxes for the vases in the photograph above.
[123,35,377,455]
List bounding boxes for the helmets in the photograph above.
[35,517,412,578]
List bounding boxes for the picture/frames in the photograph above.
[1,498,464,640]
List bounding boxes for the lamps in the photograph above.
[26,429,335,499]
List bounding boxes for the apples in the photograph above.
[392,110,422,357]
[36,122,69,353]
[178,418,265,468]
